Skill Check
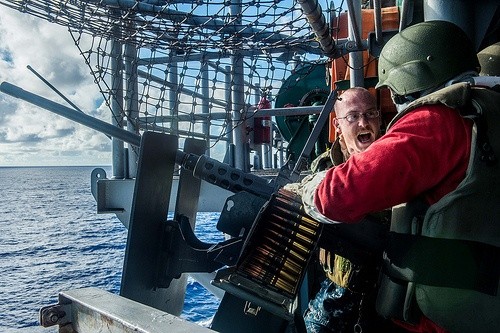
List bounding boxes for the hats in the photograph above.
[376,20,481,95]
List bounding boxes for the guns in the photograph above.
[0,80,337,324]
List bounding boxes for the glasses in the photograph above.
[336,110,379,122]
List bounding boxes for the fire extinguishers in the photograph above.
[252,92,273,144]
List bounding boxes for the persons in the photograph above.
[281,21,499,333]
[302,85,390,333]
